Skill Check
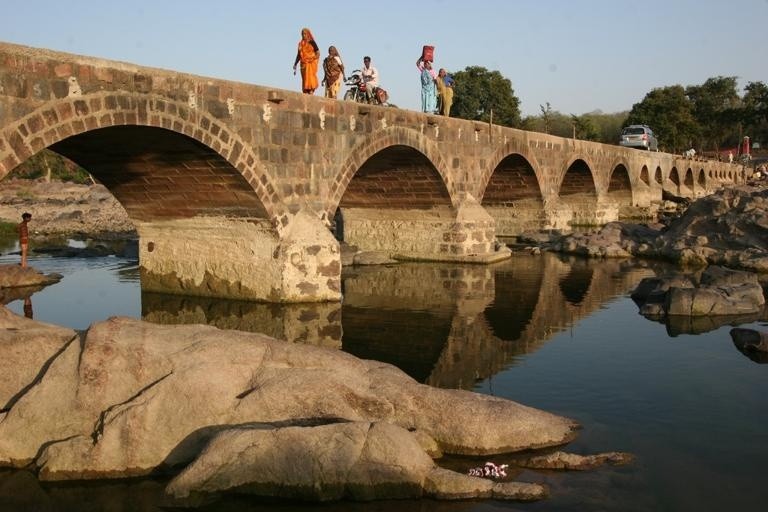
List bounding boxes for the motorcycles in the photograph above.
[343,72,391,107]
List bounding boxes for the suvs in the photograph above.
[620,123,660,153]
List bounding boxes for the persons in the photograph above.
[23,296,33,318]
[415,55,441,114]
[292,27,322,97]
[357,56,380,103]
[727,150,734,164]
[685,148,690,157]
[436,66,457,116]
[689,147,696,157]
[13,211,33,268]
[321,45,348,101]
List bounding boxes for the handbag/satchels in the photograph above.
[422,45,434,63]
